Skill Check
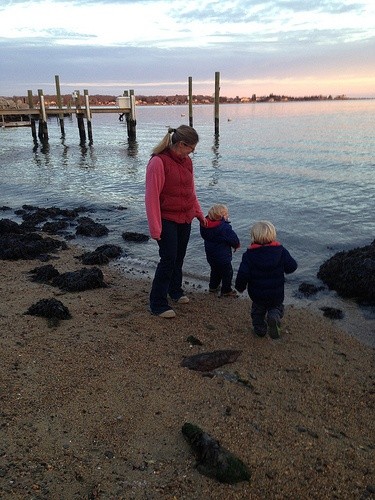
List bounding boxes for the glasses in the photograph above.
[184,142,195,153]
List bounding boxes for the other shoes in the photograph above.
[173,295,189,303]
[221,289,241,296]
[256,320,268,336]
[209,284,222,292]
[268,319,280,338]
[159,309,176,318]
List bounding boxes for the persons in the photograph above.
[145,125,208,319]
[234,221,298,339]
[200,204,240,296]
